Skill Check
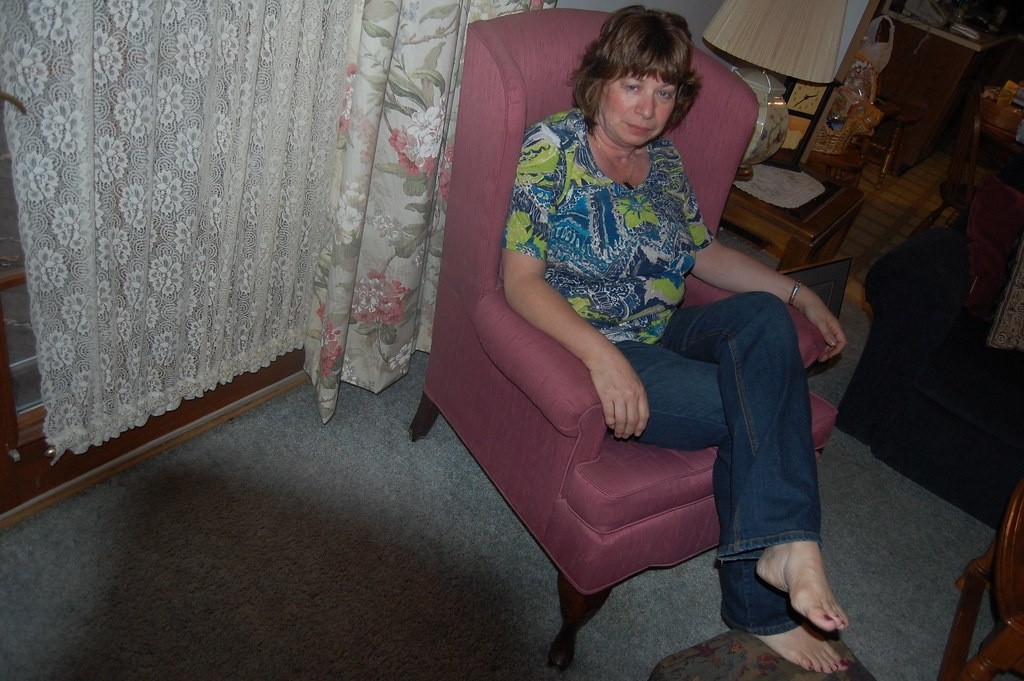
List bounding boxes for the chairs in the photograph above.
[904,86,981,242]
[410,8,840,672]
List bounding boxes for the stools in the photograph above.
[862,106,924,181]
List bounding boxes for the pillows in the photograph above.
[984,232,1024,355]
[966,176,1024,319]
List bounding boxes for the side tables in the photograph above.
[719,156,864,272]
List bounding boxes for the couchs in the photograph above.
[835,227,1024,530]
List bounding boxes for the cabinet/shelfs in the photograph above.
[865,0,1017,175]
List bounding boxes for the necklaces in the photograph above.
[595,139,635,191]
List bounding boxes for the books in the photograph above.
[952,22,981,41]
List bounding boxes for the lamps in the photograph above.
[701,0,847,181]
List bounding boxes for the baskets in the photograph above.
[810,54,884,156]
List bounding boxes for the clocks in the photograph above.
[768,76,844,173]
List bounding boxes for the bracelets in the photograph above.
[789,280,801,303]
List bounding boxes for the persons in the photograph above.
[501,4,854,675]
